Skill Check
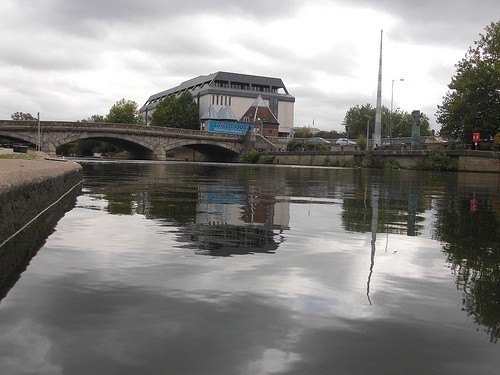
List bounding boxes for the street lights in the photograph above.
[341,122,349,146]
[390,78,405,145]
[365,114,372,151]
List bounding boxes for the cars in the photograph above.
[335,137,357,146]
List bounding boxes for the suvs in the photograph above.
[307,137,332,148]
